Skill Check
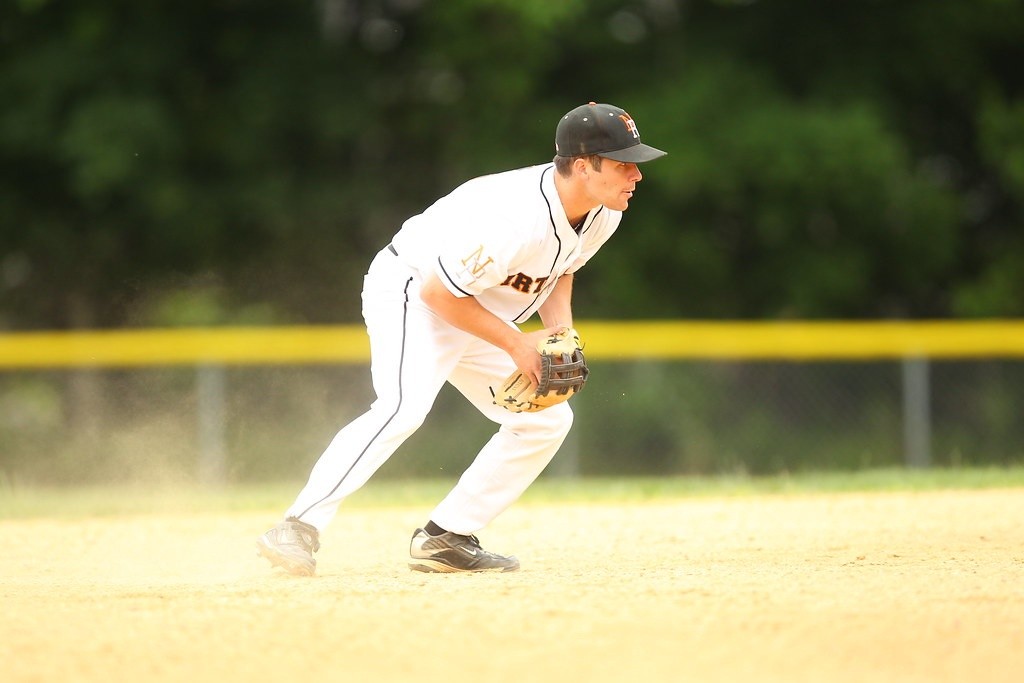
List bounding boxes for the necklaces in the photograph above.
[574,218,585,230]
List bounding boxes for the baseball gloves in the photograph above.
[487,323,592,416]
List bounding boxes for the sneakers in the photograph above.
[254,516,320,576]
[409,527,520,574]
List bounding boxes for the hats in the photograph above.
[555,102,668,163]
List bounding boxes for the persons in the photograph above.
[254,101,668,577]
